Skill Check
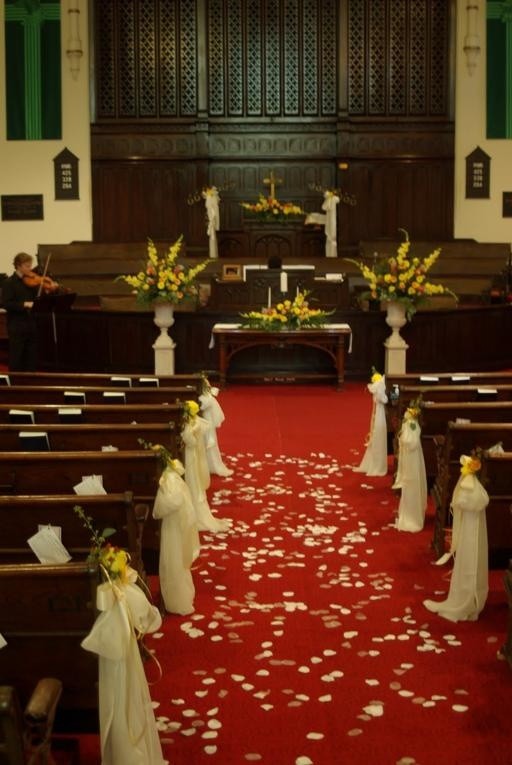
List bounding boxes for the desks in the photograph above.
[213,324,351,391]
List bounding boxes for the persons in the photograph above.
[4,252,63,374]
[266,254,282,269]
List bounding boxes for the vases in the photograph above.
[384,304,410,374]
[152,301,178,374]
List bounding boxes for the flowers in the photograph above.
[371,367,382,385]
[323,190,335,197]
[237,288,336,332]
[343,227,460,322]
[113,234,210,310]
[238,193,307,217]
[203,185,218,198]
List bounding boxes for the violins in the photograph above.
[22,271,73,293]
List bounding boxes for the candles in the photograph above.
[297,286,299,296]
[268,287,272,307]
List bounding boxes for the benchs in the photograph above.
[384,372,512,657]
